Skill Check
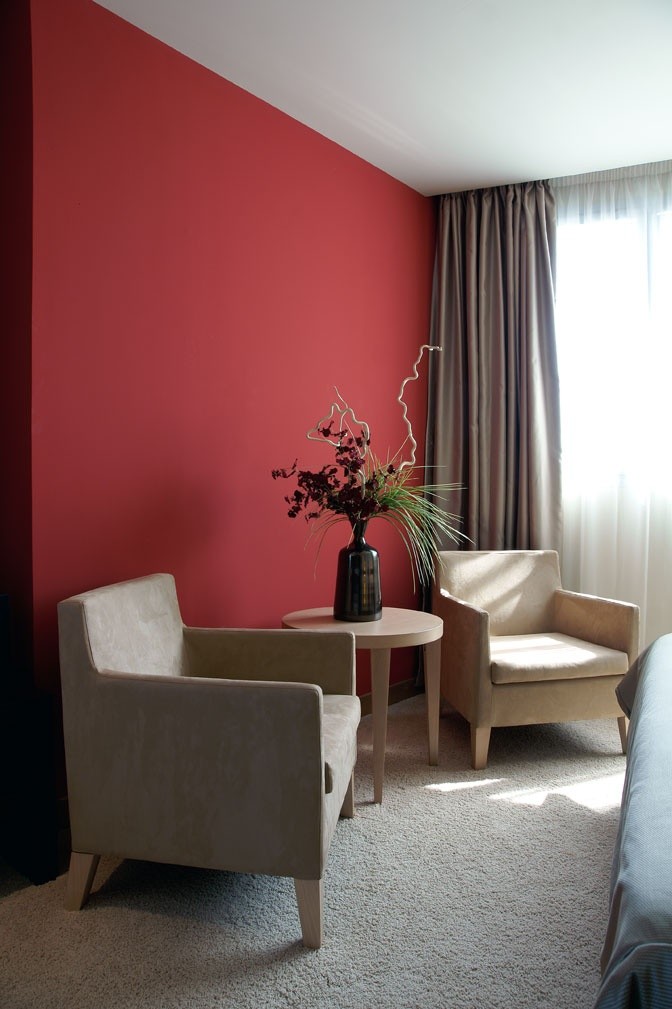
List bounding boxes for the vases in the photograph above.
[335,517,383,622]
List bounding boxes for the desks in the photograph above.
[281,606,444,805]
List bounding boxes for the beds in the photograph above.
[587,631,672,1009]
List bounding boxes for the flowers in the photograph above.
[262,340,461,591]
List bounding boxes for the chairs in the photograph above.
[56,572,362,951]
[429,549,641,772]
